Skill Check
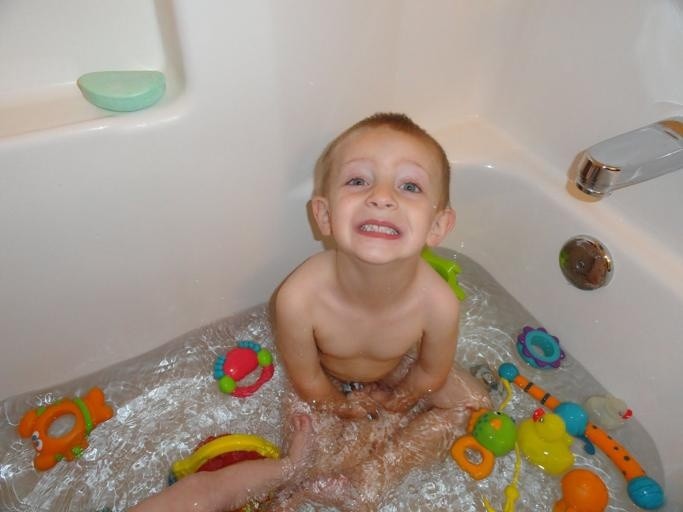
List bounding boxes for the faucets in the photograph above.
[575,118,683,197]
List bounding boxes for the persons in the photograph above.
[127,412,314,512]
[264,112,490,512]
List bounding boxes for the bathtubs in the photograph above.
[0,124,683,512]
